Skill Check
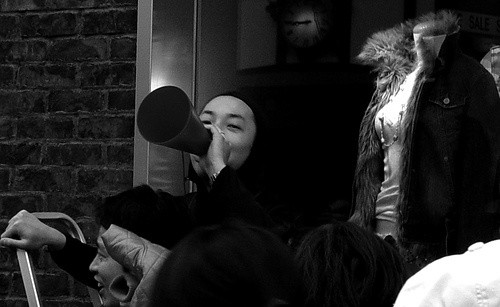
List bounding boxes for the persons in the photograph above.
[1,91,500,307]
[350,13,500,285]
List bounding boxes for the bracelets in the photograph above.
[210,166,228,184]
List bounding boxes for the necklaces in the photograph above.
[379,99,405,148]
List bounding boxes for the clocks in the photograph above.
[264,0,353,63]
